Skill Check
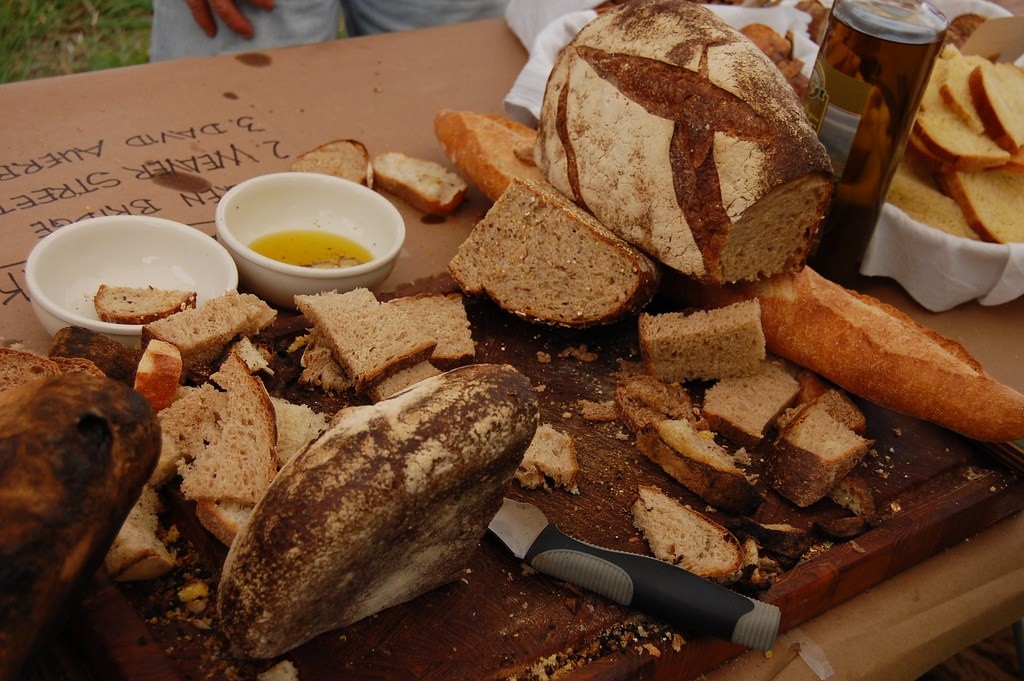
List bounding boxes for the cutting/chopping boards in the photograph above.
[9,269,1023,681]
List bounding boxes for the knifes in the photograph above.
[488,498,780,650]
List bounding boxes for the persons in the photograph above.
[148,0,511,63]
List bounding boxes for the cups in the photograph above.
[801,0,948,293]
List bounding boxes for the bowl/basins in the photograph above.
[215,172,406,310]
[26,215,239,347]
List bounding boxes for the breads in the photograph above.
[0,0,1024,681]
